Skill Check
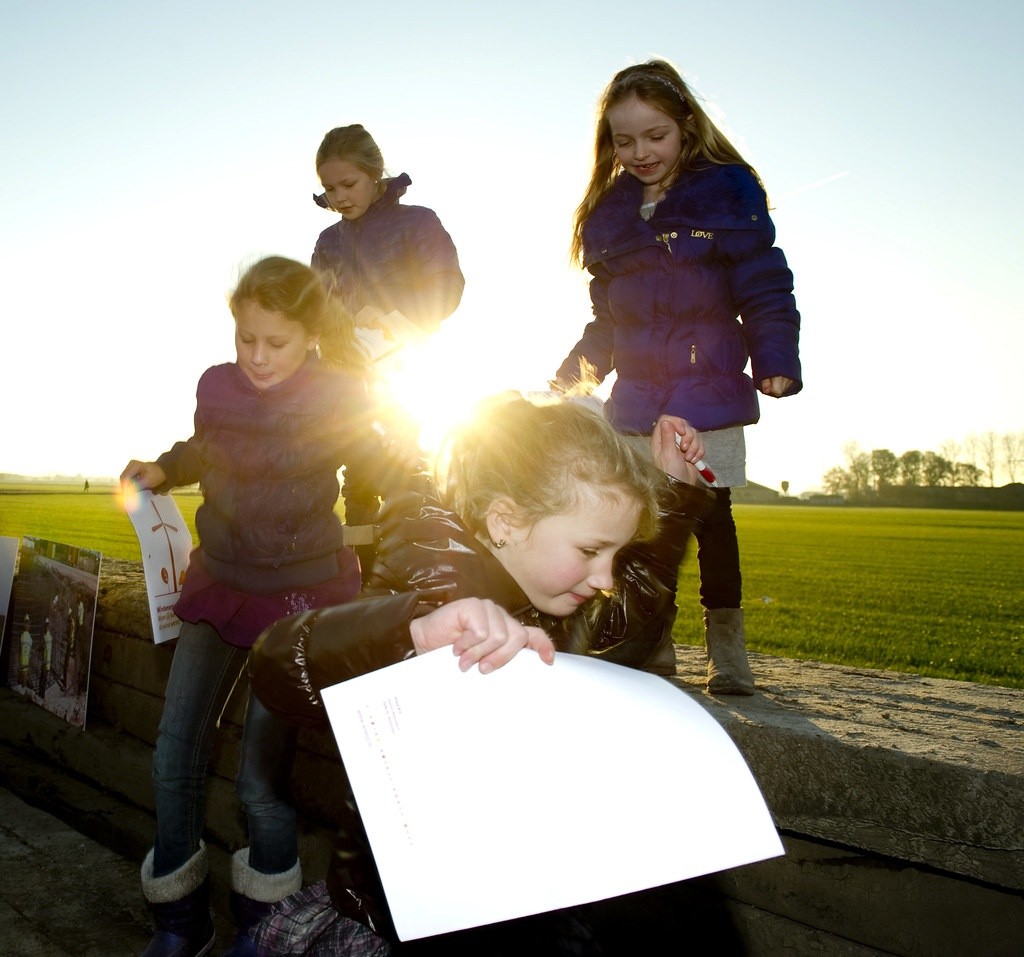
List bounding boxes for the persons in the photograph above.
[311,125,467,585]
[550,61,803,696]
[17,592,84,698]
[119,257,380,956]
[245,395,717,957]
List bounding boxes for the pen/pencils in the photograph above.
[674,431,719,488]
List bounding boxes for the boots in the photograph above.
[225,846,302,957]
[636,604,679,675]
[340,522,374,591]
[702,607,756,695]
[140,838,216,957]
